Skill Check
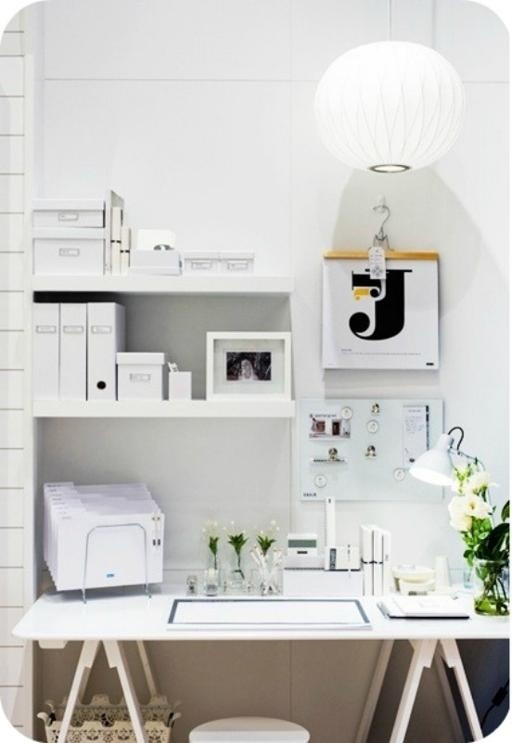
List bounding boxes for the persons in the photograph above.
[237,359,258,380]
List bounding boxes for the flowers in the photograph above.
[449,467,509,568]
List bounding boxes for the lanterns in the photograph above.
[313,41,464,174]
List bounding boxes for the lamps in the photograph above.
[408,426,494,508]
[315,2,464,174]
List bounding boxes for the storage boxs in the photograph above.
[116,351,165,404]
[33,233,110,285]
[33,199,104,227]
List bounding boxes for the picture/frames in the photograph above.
[205,331,292,403]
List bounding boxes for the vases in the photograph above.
[474,561,509,615]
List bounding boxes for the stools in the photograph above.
[187,716,310,743]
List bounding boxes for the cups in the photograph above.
[399,575,428,597]
[168,371,193,403]
[185,568,285,597]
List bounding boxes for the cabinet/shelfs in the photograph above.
[27,281,299,418]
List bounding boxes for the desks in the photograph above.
[12,587,509,743]
